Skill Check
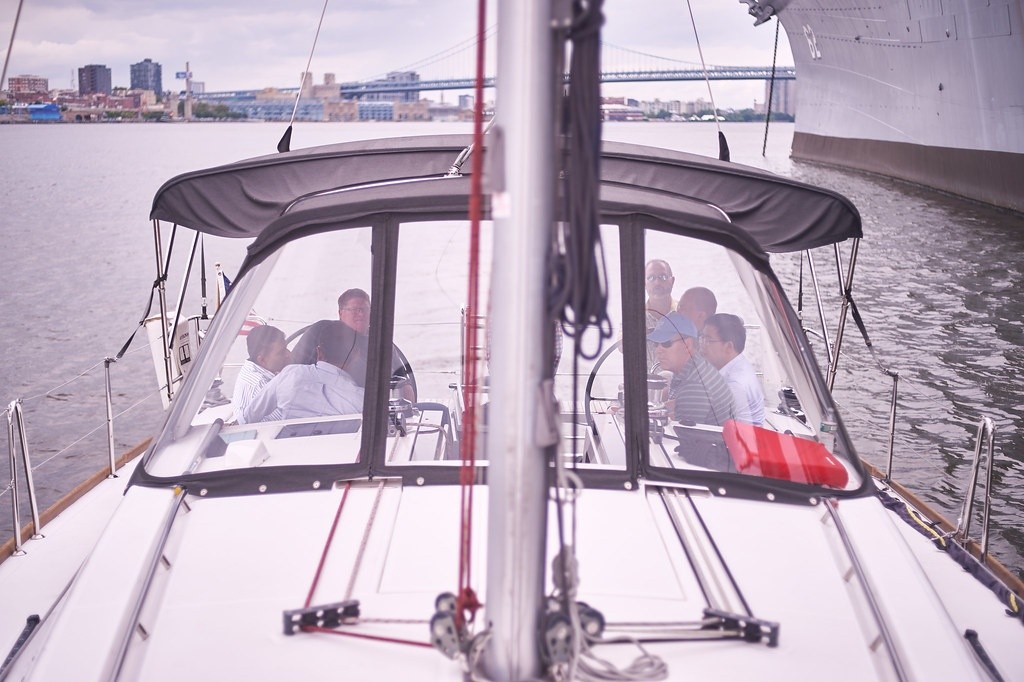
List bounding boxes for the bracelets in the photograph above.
[651,362,660,373]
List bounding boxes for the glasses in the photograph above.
[341,307,370,316]
[699,337,721,346]
[653,336,689,349]
[646,274,673,282]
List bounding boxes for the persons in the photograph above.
[646,310,738,427]
[233,325,290,425]
[699,313,765,427]
[619,259,681,374]
[243,322,366,424]
[282,287,371,388]
[676,287,717,357]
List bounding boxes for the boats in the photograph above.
[739,0,1024,218]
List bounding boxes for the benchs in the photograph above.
[417,397,610,423]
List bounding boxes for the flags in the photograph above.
[222,272,257,316]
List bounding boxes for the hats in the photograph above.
[647,311,698,343]
